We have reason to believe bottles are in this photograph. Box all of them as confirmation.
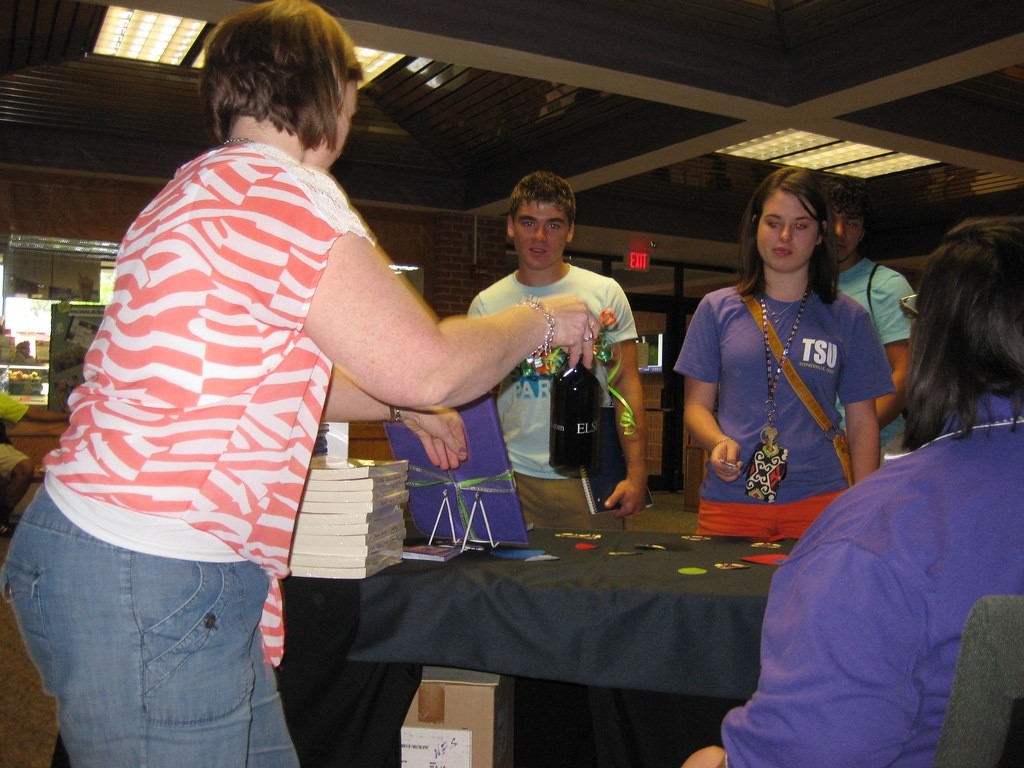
[550,346,601,471]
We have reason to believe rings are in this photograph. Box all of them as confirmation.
[584,330,593,342]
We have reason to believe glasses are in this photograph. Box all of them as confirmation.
[898,294,920,319]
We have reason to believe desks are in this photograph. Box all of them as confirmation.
[47,528,802,768]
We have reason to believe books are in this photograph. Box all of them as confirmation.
[580,406,652,515]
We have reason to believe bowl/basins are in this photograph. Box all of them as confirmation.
[9,379,42,396]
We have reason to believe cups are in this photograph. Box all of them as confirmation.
[36,340,49,361]
[0,334,16,362]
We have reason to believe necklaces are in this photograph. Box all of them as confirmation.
[764,291,804,324]
[222,138,254,145]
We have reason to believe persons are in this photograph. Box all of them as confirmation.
[681,218,1024,768]
[820,177,917,432]
[674,167,896,539]
[0,389,69,538]
[0,0,599,768]
[465,173,652,532]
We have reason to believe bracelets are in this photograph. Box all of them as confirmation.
[710,438,735,455]
[389,405,400,426]
[517,300,554,359]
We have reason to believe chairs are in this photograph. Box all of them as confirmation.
[930,594,1024,768]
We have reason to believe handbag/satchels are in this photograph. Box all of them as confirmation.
[829,428,855,490]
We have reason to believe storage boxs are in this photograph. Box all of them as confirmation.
[7,378,41,394]
[401,666,515,768]
